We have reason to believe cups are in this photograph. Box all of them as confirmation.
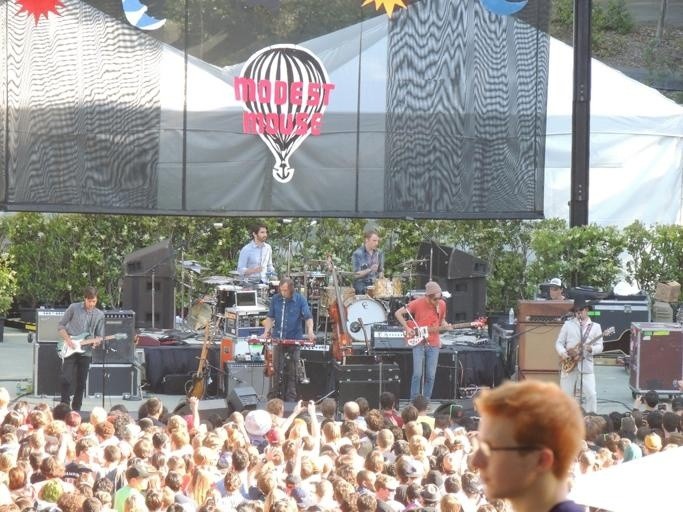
[317,265,321,274]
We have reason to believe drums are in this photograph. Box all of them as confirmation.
[343,293,389,343]
[291,270,326,290]
[368,277,403,299]
[215,284,243,305]
[191,301,214,329]
[320,286,355,318]
[268,280,281,295]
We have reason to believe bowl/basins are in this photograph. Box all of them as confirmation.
[250,344,263,355]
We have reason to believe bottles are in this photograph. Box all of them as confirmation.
[383,277,391,297]
[374,271,386,297]
[366,285,375,298]
[391,275,401,297]
[175,314,183,324]
[508,307,514,324]
[264,271,277,282]
[15,381,21,395]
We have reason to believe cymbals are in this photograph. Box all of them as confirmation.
[180,260,214,272]
[395,259,429,266]
[199,275,233,284]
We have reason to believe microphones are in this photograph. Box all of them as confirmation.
[578,316,584,326]
[101,302,107,309]
[282,233,296,240]
[395,299,408,307]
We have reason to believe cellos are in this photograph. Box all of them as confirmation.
[323,251,353,361]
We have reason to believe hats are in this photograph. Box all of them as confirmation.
[643,432,663,451]
[619,417,637,434]
[567,294,593,313]
[539,277,567,291]
[245,409,273,436]
[424,281,443,296]
[125,464,156,480]
[622,443,642,464]
[419,484,441,503]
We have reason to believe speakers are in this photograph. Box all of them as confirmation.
[125,239,176,276]
[91,309,136,365]
[122,276,175,329]
[417,280,487,324]
[372,347,413,399]
[36,308,67,344]
[295,344,335,401]
[416,242,489,280]
[430,351,457,402]
[223,361,274,402]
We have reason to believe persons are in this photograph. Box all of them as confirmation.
[554,295,604,413]
[0,378,683,512]
[237,224,278,286]
[548,278,566,300]
[351,222,384,295]
[394,282,446,407]
[263,279,315,401]
[57,288,105,410]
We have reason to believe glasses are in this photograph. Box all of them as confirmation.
[382,487,398,493]
[473,433,542,457]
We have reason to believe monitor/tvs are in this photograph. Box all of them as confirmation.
[235,290,258,307]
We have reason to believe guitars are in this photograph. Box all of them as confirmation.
[406,317,489,348]
[558,326,616,372]
[57,334,128,360]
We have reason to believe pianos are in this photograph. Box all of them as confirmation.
[516,299,574,373]
[249,338,315,347]
